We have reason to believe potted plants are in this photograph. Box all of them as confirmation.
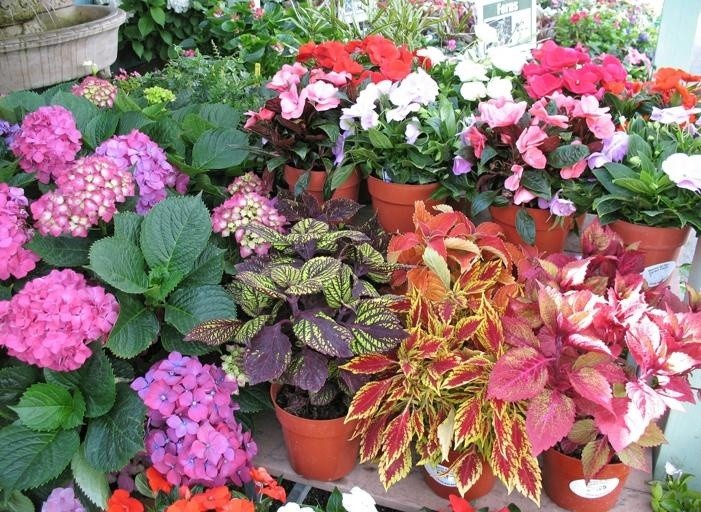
[171,198,413,481]
[483,218,701,512]
[340,199,543,507]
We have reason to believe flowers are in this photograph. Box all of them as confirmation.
[241,61,358,201]
[338,67,472,184]
[586,107,701,230]
[450,91,616,246]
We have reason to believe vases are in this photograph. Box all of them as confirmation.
[490,206,566,259]
[283,165,359,208]
[367,174,447,232]
[576,213,689,268]
